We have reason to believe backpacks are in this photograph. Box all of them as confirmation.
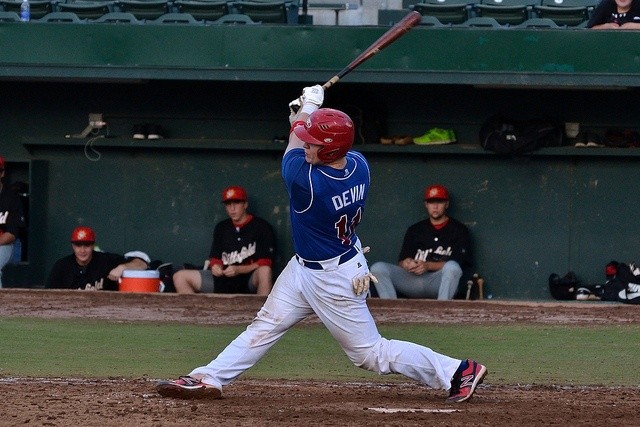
[549,269,593,299]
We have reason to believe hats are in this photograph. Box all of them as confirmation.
[423,185,449,201]
[221,186,247,202]
[70,227,95,243]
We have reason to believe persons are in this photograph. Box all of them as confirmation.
[155,83,487,403]
[171,184,273,293]
[0,155,22,275]
[585,0,640,30]
[44,226,148,291]
[369,184,472,302]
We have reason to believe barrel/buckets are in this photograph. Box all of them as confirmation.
[117,270,160,292]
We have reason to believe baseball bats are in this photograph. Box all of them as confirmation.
[202,260,211,271]
[289,11,423,115]
[466,280,474,301]
[472,273,479,301]
[476,278,486,299]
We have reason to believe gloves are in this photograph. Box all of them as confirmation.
[353,273,379,295]
[363,246,370,254]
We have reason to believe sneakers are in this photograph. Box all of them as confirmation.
[448,130,455,142]
[573,132,587,147]
[148,122,169,140]
[445,357,488,403]
[132,122,147,139]
[155,375,222,400]
[395,135,412,146]
[587,134,606,147]
[380,135,398,144]
[412,128,451,146]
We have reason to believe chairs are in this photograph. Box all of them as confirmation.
[451,17,509,29]
[415,15,452,28]
[510,18,567,29]
[145,12,205,26]
[205,13,262,25]
[87,12,145,25]
[0,11,20,22]
[30,11,88,24]
[568,19,590,29]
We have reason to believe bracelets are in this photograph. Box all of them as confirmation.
[289,121,308,135]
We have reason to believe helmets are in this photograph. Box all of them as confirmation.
[293,108,355,162]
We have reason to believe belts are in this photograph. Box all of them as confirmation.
[295,245,360,269]
